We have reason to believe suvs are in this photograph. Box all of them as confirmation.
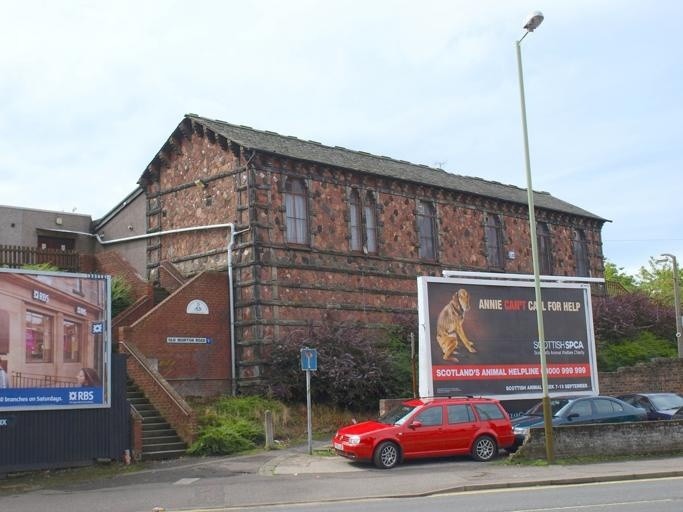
[332,394,513,469]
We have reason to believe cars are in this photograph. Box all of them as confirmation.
[614,392,683,421]
[505,394,649,452]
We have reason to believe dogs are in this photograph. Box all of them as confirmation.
[436,288,477,363]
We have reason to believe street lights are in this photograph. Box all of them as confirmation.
[656,252,683,360]
[513,10,554,465]
